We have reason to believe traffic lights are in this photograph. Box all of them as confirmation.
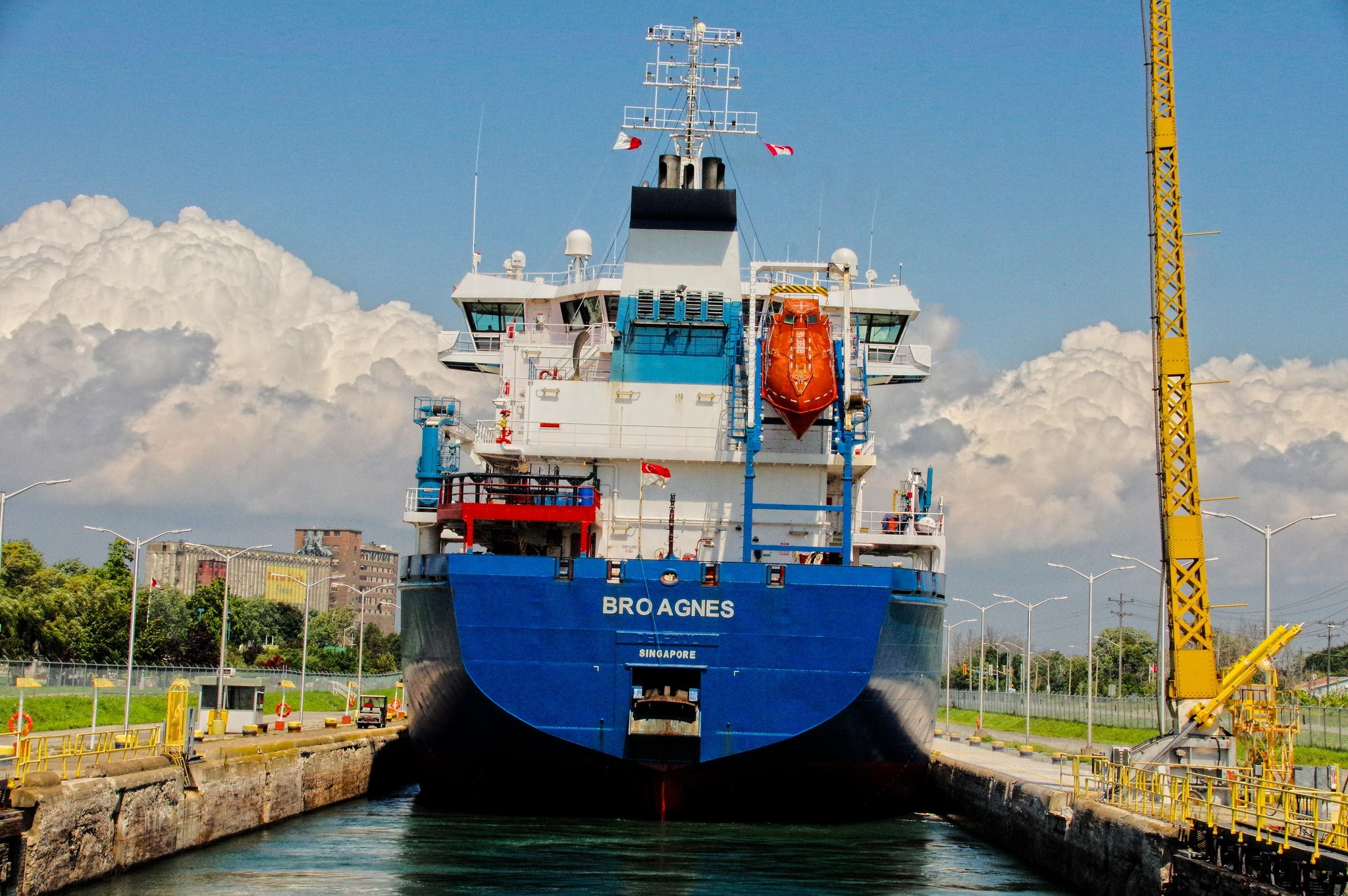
[963,664,966,675]
[1002,665,1005,673]
[1010,666,1013,677]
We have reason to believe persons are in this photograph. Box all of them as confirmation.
[487,325,495,331]
[364,699,374,707]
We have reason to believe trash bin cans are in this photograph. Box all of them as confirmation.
[545,496,555,506]
[566,492,573,506]
[578,487,594,505]
[556,493,566,506]
[534,496,541,505]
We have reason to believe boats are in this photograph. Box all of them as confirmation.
[761,297,838,440]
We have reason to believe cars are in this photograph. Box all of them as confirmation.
[356,695,388,729]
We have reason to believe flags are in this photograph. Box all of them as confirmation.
[613,131,643,150]
[642,463,672,488]
[152,577,161,589]
[765,142,794,156]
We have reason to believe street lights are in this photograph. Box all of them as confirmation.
[0,478,72,533]
[1030,652,1051,695]
[1201,508,1338,705]
[332,582,395,710]
[995,643,1009,693]
[82,525,192,734]
[992,592,1069,746]
[183,541,274,720]
[1069,645,1099,697]
[344,626,356,647]
[272,571,348,723]
[1004,641,1025,693]
[1094,636,1122,699]
[1047,562,1136,749]
[1109,552,1220,735]
[1050,649,1072,695]
[1020,654,1039,693]
[943,618,980,735]
[950,597,1015,732]
[982,643,1000,693]
[380,601,405,711]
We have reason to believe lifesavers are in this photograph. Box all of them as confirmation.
[390,698,402,709]
[976,716,980,730]
[275,702,291,717]
[349,695,355,708]
[7,712,33,736]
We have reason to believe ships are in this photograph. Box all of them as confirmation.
[397,15,948,810]
